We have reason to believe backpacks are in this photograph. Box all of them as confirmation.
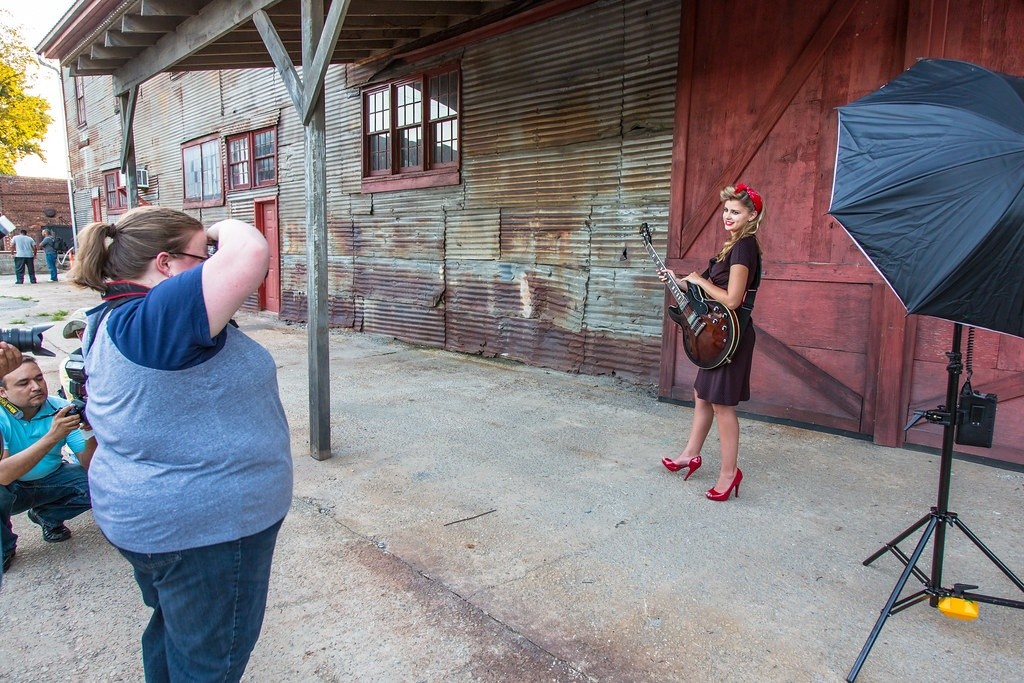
[47,236,63,250]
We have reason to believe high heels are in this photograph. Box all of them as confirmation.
[705,467,743,501]
[662,456,702,481]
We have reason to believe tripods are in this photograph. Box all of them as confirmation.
[845,322,1024,683]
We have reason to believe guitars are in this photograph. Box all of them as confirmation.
[637,219,741,370]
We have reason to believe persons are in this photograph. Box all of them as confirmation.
[67,205,293,683]
[11,230,37,283]
[656,183,764,501]
[0,307,98,574]
[40,228,61,282]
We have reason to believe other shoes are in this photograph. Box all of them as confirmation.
[31,282,37,284]
[47,279,58,283]
[15,282,23,284]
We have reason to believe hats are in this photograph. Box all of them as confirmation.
[63,306,96,339]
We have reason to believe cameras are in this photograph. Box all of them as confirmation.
[65,360,89,426]
[0,326,56,357]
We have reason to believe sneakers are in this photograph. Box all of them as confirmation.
[0,549,16,573]
[28,510,71,541]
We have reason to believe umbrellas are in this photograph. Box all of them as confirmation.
[826,59,1024,683]
